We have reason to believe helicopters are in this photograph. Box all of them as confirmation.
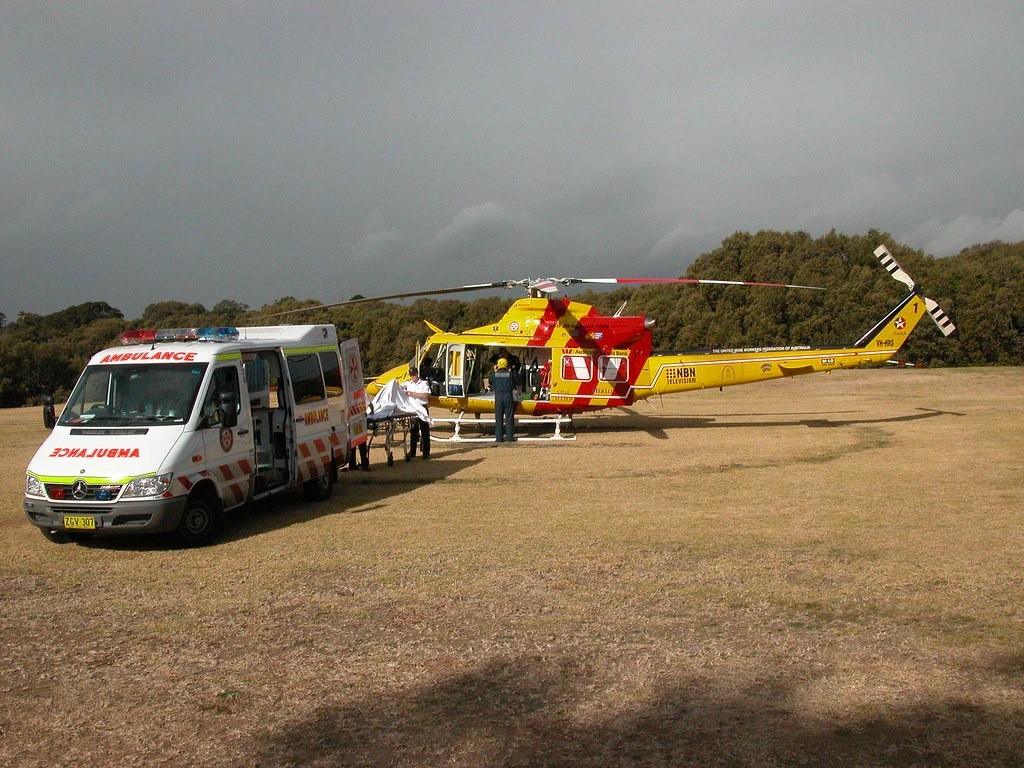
[244,238,956,446]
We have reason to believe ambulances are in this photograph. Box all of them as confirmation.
[21,323,370,548]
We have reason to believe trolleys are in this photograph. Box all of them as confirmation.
[365,408,426,467]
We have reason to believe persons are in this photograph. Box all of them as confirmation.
[401,368,432,459]
[489,347,521,442]
[349,393,375,471]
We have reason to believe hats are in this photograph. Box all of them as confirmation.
[409,367,418,374]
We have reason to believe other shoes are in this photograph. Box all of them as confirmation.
[495,440,504,442]
[508,438,517,441]
[422,454,430,459]
[407,453,415,457]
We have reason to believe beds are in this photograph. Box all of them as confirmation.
[366,412,418,465]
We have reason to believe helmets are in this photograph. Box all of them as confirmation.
[497,358,509,369]
